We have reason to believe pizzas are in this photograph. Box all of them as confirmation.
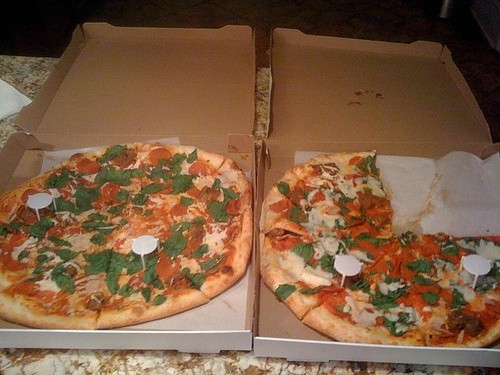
[259,150,500,348]
[0,141,252,330]
